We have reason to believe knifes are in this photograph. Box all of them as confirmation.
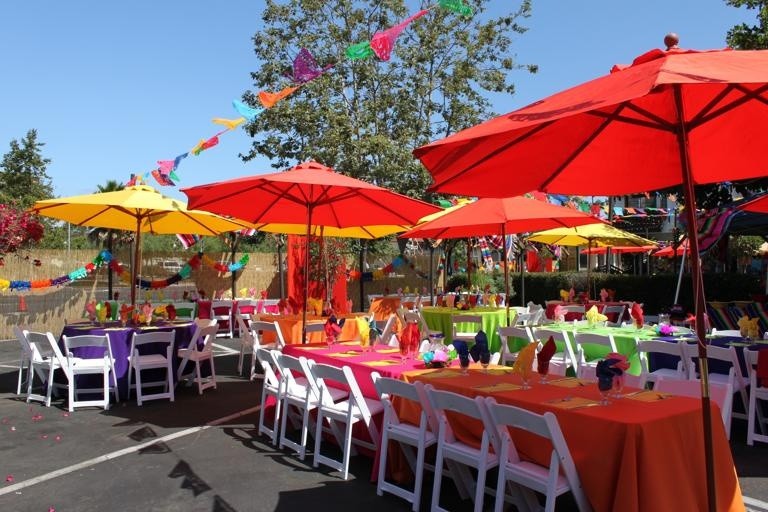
[627,387,651,397]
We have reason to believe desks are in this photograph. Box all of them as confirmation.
[259,311,369,328]
[372,293,443,304]
[199,300,280,319]
[544,299,633,312]
[445,291,506,301]
[510,321,692,346]
[399,362,716,436]
[420,305,516,321]
[65,320,195,336]
[651,334,767,352]
[281,343,476,384]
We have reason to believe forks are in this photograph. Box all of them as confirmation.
[656,393,680,401]
[548,395,572,405]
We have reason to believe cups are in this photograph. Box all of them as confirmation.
[740,326,749,343]
[748,329,757,348]
[325,336,334,351]
[121,315,127,327]
[359,334,369,353]
[144,314,152,326]
[408,341,419,363]
[520,364,532,391]
[480,351,491,375]
[89,311,96,325]
[398,343,411,366]
[537,362,551,384]
[611,374,623,398]
[457,354,472,377]
[597,377,610,406]
[370,333,376,351]
[552,314,560,328]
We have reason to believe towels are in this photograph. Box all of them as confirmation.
[421,285,428,298]
[166,303,177,321]
[514,341,540,379]
[240,288,249,296]
[468,292,478,307]
[143,304,153,323]
[630,303,644,325]
[749,318,758,337]
[489,295,496,308]
[585,305,608,327]
[551,305,567,322]
[569,288,574,301]
[248,287,256,296]
[452,339,471,368]
[594,359,621,390]
[355,314,370,348]
[330,316,345,338]
[118,303,132,322]
[171,290,180,302]
[144,289,152,303]
[214,287,226,303]
[261,290,268,299]
[403,285,411,295]
[369,320,378,345]
[322,321,333,342]
[85,301,97,322]
[738,316,750,335]
[98,306,108,323]
[560,290,568,303]
[413,287,419,297]
[157,290,166,302]
[538,335,557,374]
[495,294,503,306]
[445,293,457,307]
[599,288,607,303]
[470,331,491,366]
[105,302,112,319]
[395,287,402,296]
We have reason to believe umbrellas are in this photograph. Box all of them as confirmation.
[415,201,483,293]
[31,178,247,313]
[525,224,659,301]
[178,159,446,344]
[736,190,767,215]
[227,214,416,314]
[651,237,701,272]
[397,197,613,329]
[412,33,768,512]
[579,245,658,273]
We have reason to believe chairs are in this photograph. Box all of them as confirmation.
[400,296,419,313]
[175,323,220,396]
[368,294,384,305]
[402,309,444,343]
[637,340,685,392]
[63,332,120,413]
[498,326,535,365]
[261,299,281,315]
[420,296,433,307]
[370,371,467,511]
[532,360,567,376]
[559,305,585,321]
[22,329,76,408]
[233,299,258,338]
[306,324,326,331]
[744,346,768,446]
[456,351,501,364]
[256,349,325,445]
[575,363,647,392]
[486,394,593,512]
[424,382,530,512]
[236,314,253,377]
[306,358,386,478]
[531,326,577,375]
[711,327,742,338]
[651,375,733,441]
[127,330,175,406]
[210,299,233,339]
[380,313,397,345]
[174,302,196,323]
[273,349,350,461]
[249,320,285,384]
[13,326,60,398]
[603,305,626,326]
[682,340,752,421]
[451,314,483,342]
[572,329,619,381]
